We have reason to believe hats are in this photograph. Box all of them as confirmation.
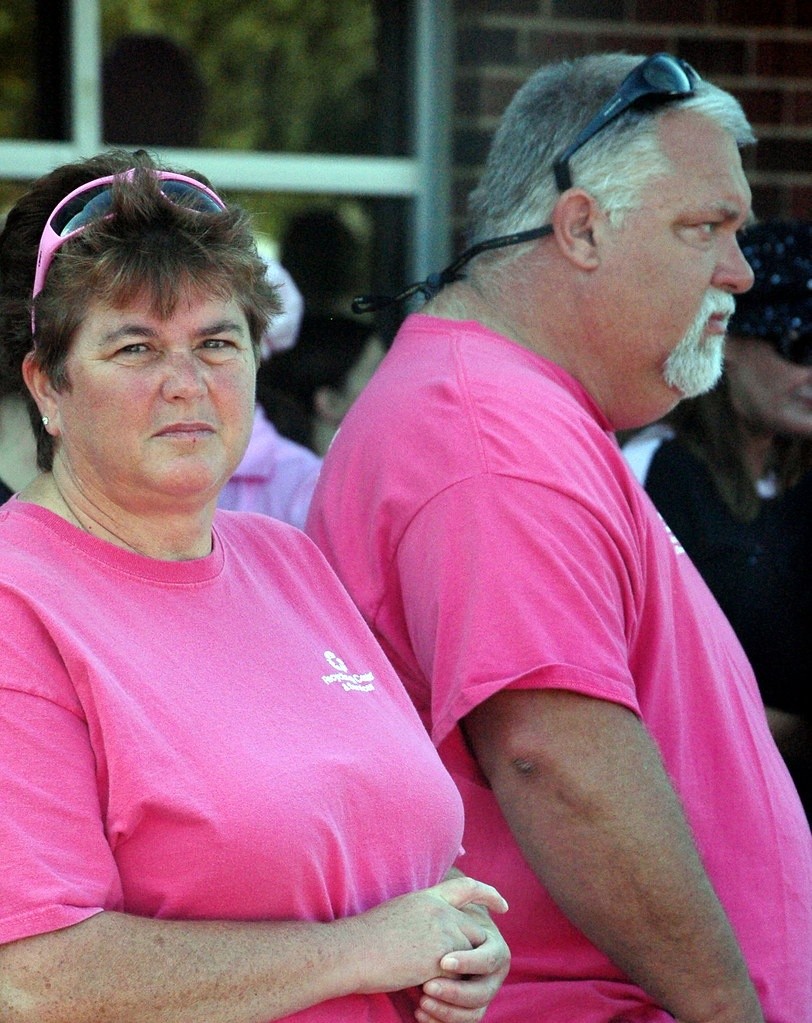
[727,220,812,341]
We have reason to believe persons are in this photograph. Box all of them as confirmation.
[0,147,513,1023]
[303,49,812,1023]
[3,34,812,829]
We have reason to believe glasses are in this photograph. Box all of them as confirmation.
[767,337,812,366]
[32,168,231,349]
[553,54,700,196]
